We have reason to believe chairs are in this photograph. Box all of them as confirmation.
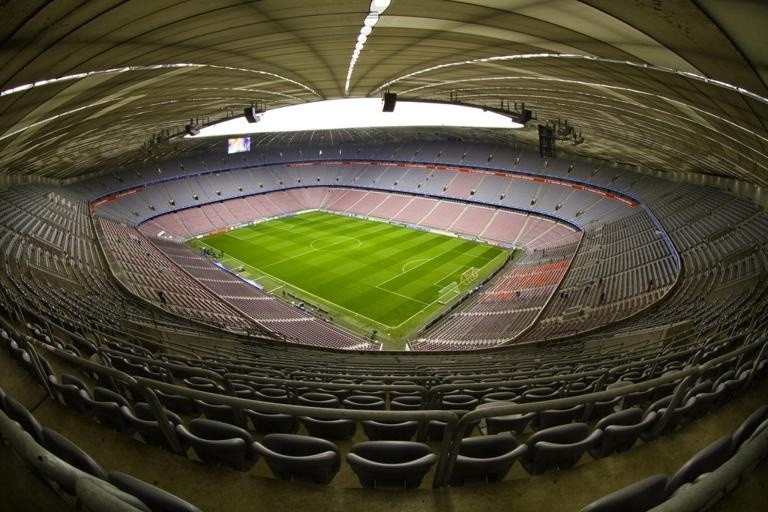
[0,140,768,511]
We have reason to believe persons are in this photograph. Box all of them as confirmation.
[157,289,167,306]
[645,279,653,294]
[204,247,224,260]
[515,290,522,298]
[146,252,149,257]
[282,289,285,298]
[137,240,141,245]
[557,276,606,305]
